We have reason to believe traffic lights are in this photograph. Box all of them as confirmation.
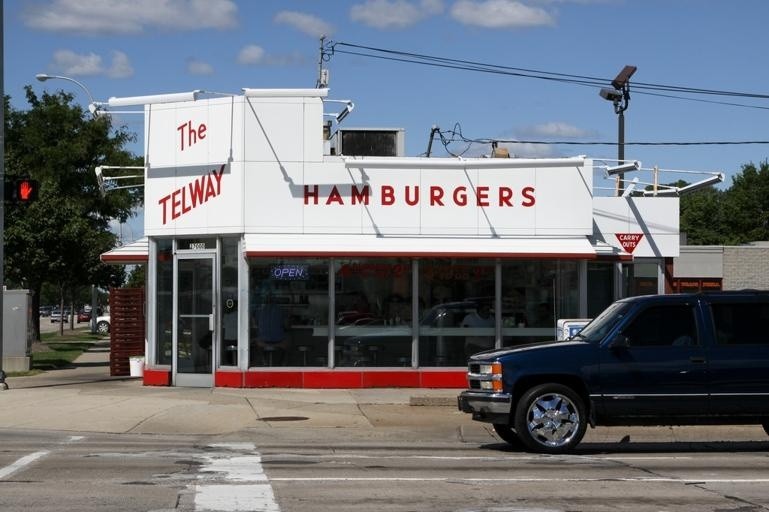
[18,179,36,199]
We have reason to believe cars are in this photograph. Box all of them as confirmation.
[77,304,103,324]
[337,297,528,367]
[38,304,74,324]
[88,316,110,333]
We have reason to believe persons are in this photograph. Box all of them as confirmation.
[459,301,496,362]
[222,302,288,363]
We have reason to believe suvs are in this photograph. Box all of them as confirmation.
[456,291,769,453]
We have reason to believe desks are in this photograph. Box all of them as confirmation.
[310,327,556,339]
[250,324,314,329]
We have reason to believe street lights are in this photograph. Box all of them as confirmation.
[36,72,94,105]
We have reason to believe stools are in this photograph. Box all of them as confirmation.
[208,344,449,366]
